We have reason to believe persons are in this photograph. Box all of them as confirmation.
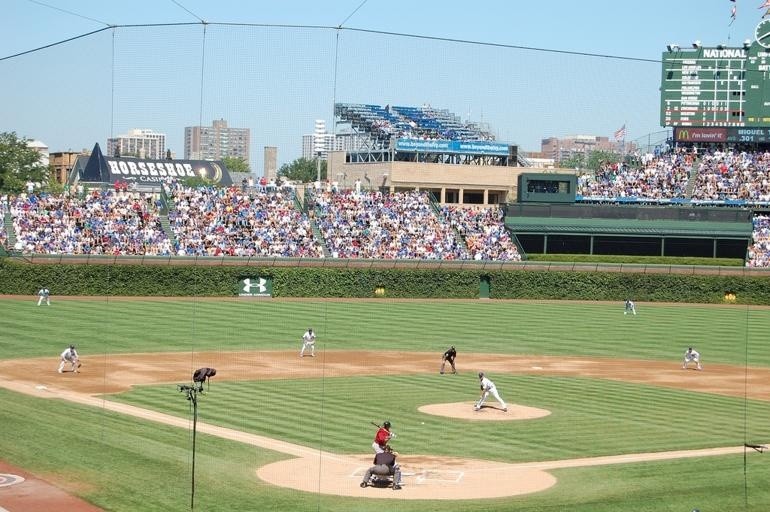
[473,372,507,411]
[1,175,522,261]
[440,345,458,374]
[372,104,496,141]
[623,299,636,315]
[37,287,50,306]
[683,348,700,369]
[300,327,317,359]
[57,345,80,374]
[360,421,403,490]
[576,142,769,266]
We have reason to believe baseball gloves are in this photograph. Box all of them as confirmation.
[392,452,398,456]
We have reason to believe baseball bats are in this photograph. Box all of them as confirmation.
[371,421,394,436]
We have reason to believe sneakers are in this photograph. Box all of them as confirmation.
[393,485,401,490]
[360,483,366,488]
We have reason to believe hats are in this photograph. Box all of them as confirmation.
[382,446,393,450]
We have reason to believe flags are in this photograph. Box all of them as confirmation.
[614,125,626,142]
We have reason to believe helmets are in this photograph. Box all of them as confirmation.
[384,421,391,428]
